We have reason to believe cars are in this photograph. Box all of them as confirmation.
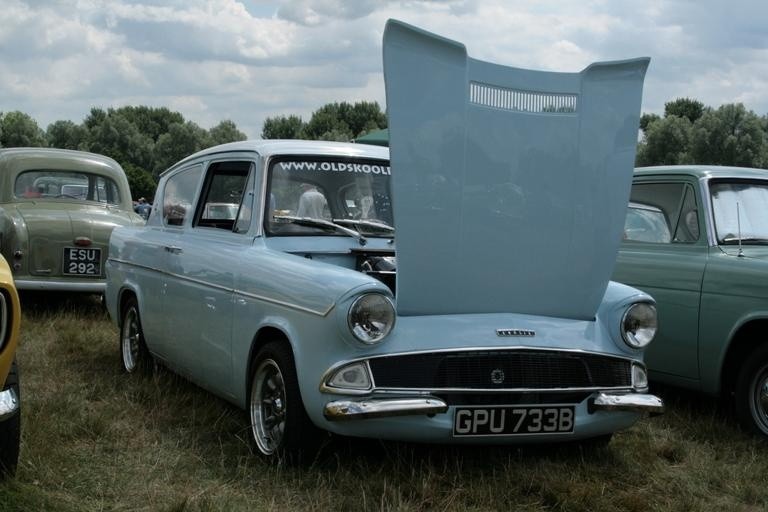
[0,147,147,294]
[611,161,767,440]
[104,16,664,468]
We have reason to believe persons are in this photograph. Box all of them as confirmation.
[295,182,327,222]
[12,180,293,225]
